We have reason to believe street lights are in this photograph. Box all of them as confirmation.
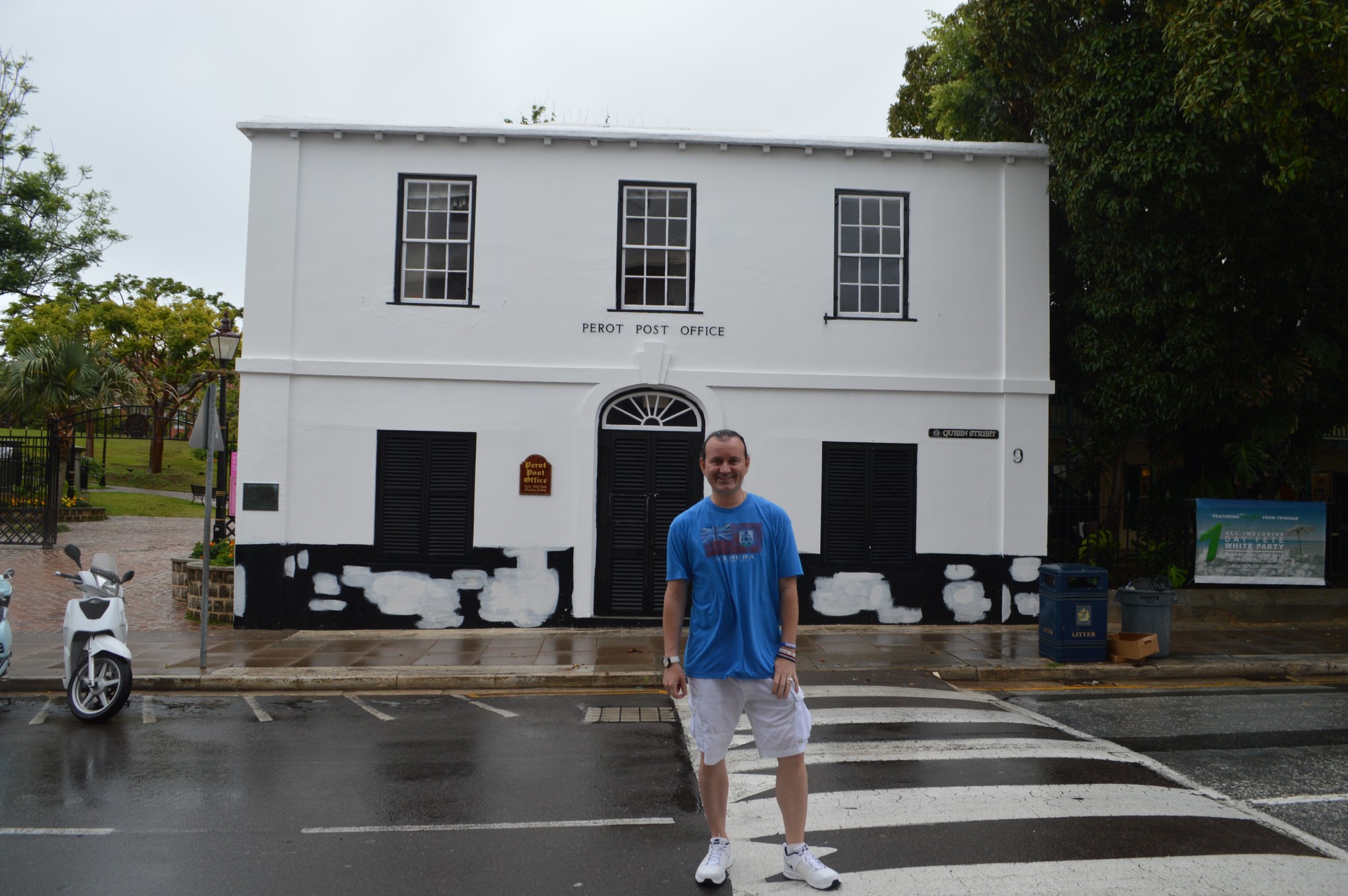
[208,308,245,544]
[99,398,115,486]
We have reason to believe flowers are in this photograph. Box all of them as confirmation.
[1,495,93,508]
[191,537,235,566]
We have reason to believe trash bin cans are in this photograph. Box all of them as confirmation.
[1115,586,1175,659]
[1038,563,1111,662]
[0,440,25,486]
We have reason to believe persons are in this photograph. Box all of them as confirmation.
[662,429,840,892]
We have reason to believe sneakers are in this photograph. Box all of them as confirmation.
[695,834,735,883]
[784,842,841,891]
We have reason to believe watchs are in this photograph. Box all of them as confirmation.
[662,656,680,667]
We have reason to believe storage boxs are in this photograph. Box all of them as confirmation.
[1107,632,1160,663]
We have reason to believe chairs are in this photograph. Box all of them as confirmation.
[190,484,229,505]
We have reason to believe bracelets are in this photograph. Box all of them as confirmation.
[780,642,796,648]
[777,648,797,663]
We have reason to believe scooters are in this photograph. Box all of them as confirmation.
[0,568,16,703]
[55,544,135,724]
